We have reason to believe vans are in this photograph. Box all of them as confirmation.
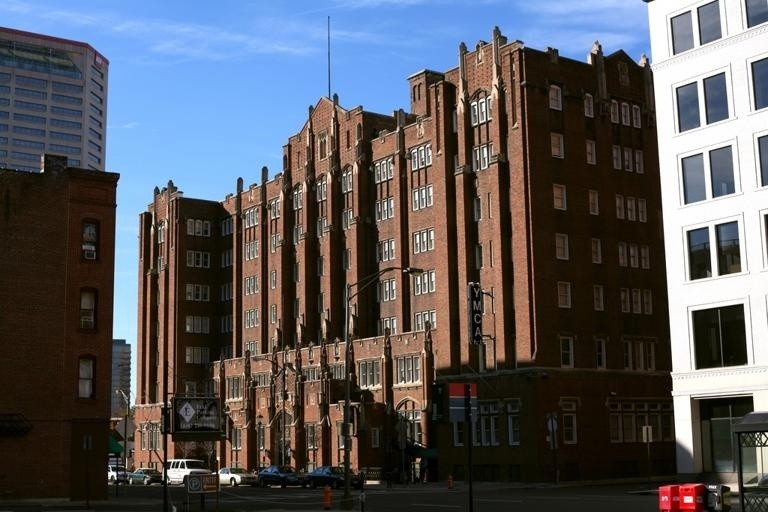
[161,458,213,485]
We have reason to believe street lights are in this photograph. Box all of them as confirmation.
[341,267,423,512]
[253,357,285,467]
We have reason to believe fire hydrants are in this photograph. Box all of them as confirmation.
[448,475,453,489]
[323,484,333,510]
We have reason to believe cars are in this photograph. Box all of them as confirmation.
[212,467,259,487]
[258,465,359,489]
[108,465,162,485]
